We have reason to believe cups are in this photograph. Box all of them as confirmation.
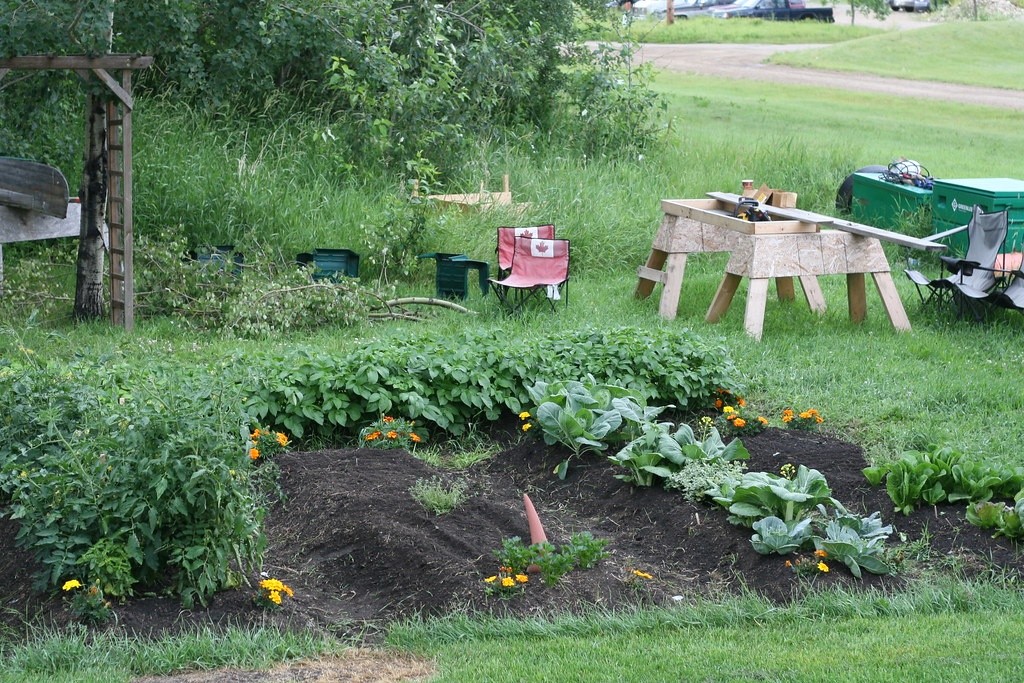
[741,180,753,199]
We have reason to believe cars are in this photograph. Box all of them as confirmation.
[889,0,930,13]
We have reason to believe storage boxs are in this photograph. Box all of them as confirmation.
[850,171,1024,261]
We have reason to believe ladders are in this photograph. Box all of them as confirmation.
[103,71,134,331]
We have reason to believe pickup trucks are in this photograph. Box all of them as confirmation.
[706,0,835,23]
[633,0,805,20]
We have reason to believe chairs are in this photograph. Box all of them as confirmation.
[494,224,556,309]
[418,252,490,304]
[903,203,1024,326]
[487,236,570,318]
[184,244,244,276]
[296,248,360,282]
